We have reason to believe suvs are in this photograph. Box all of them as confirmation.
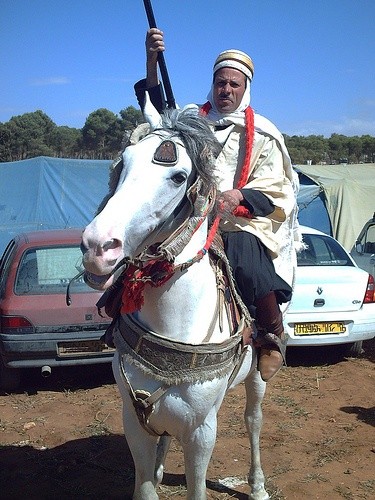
[349,212,375,277]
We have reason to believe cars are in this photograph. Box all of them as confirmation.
[1,229,115,378]
[280,225,375,356]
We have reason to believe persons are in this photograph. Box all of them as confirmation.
[101,27,295,383]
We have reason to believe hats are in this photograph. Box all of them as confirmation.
[212,49,254,83]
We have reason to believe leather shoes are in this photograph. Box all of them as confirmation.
[258,327,285,381]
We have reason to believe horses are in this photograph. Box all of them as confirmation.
[78,88,273,500]
